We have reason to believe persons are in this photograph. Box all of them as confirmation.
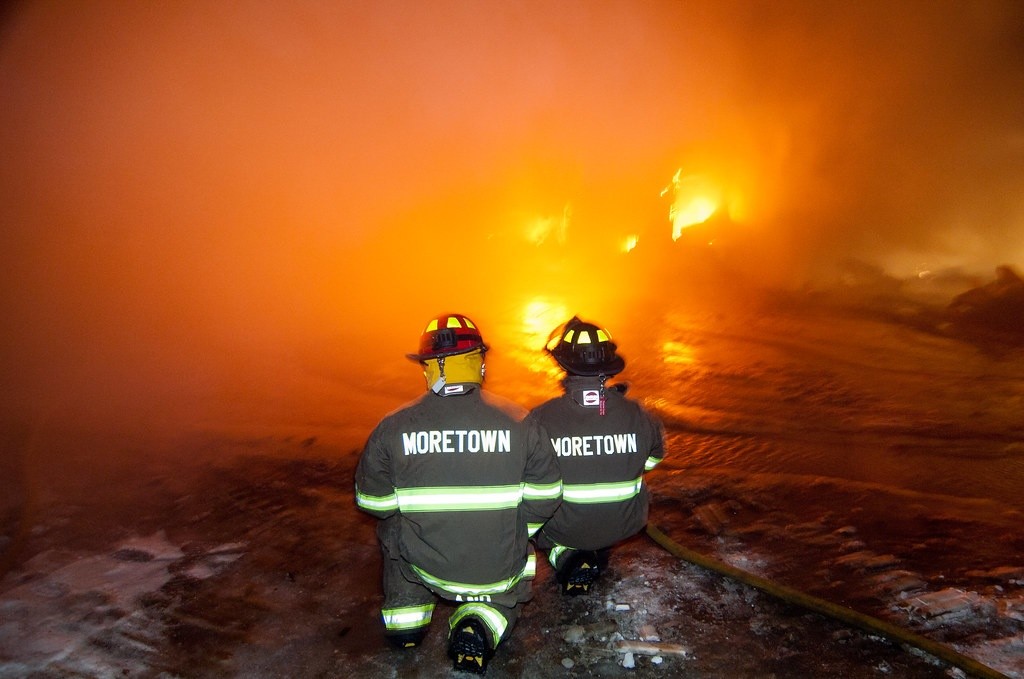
[352,313,564,678]
[522,314,665,588]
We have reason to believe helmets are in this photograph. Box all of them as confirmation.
[404,314,491,363]
[551,323,625,377]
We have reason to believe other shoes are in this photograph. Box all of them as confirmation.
[560,546,609,593]
[389,626,429,651]
[447,617,488,674]
